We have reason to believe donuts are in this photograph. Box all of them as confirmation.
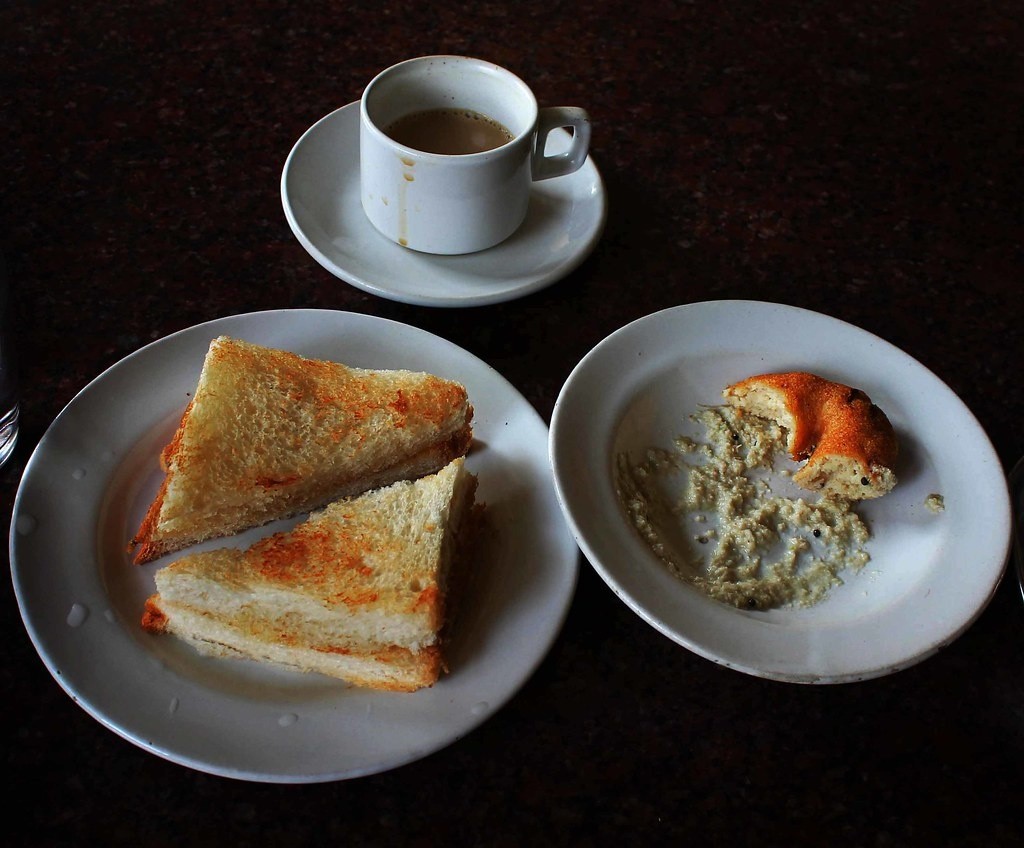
[720,371,900,502]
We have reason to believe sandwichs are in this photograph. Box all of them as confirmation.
[128,338,476,692]
